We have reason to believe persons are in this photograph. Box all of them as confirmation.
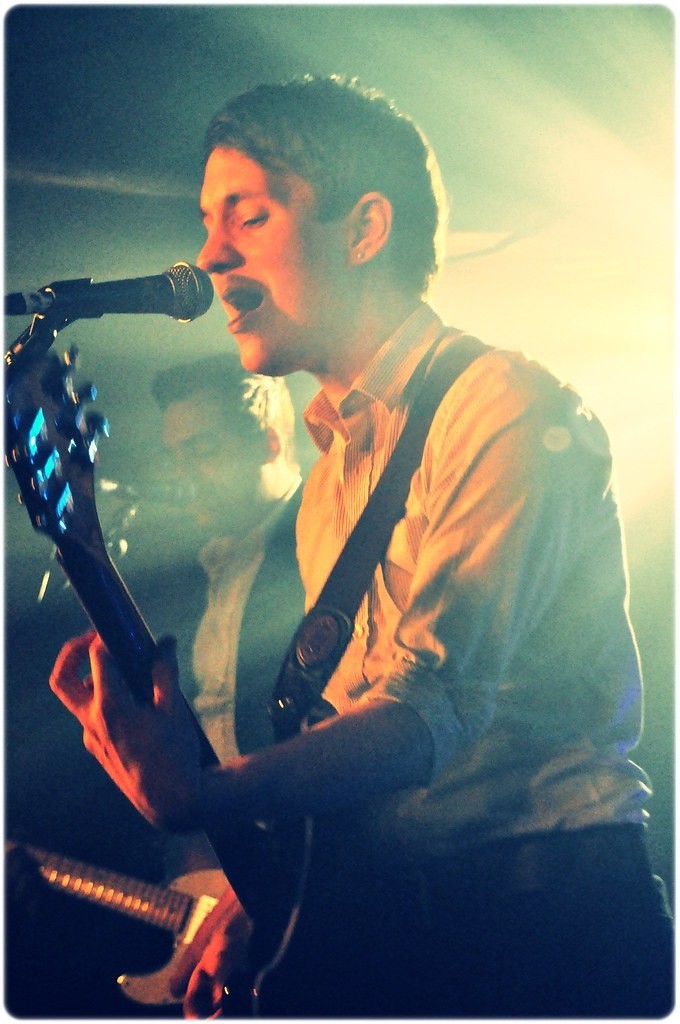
[50,71,675,1017]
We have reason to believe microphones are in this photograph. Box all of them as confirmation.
[4,261,214,324]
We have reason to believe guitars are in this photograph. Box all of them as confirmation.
[3,296,399,1020]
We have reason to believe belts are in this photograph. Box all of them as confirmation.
[348,827,649,904]
